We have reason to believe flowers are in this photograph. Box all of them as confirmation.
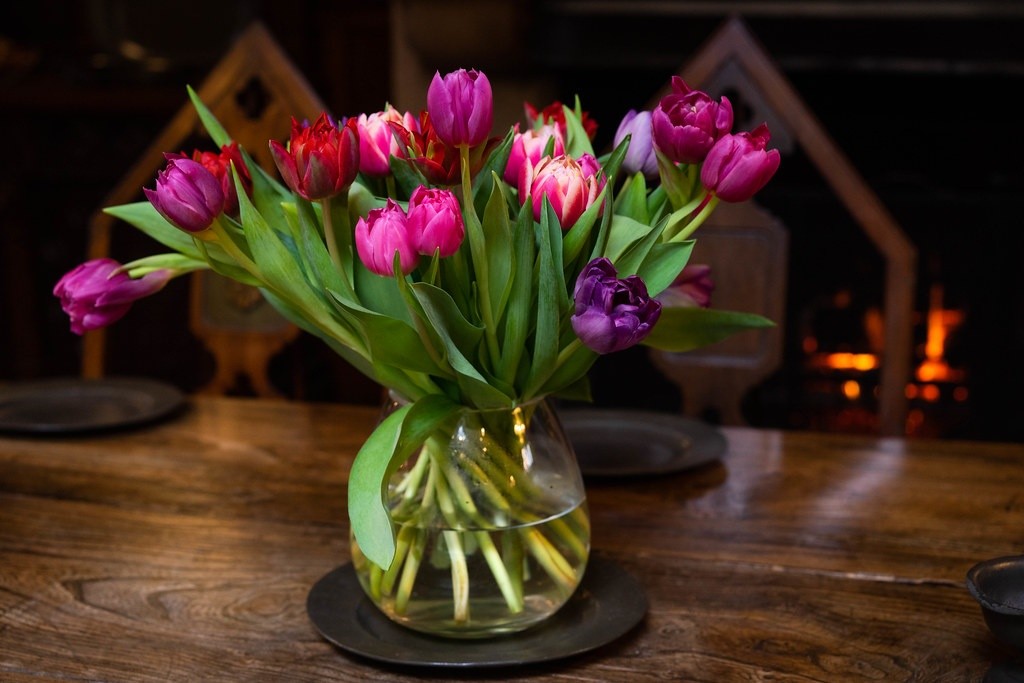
[51,67,785,625]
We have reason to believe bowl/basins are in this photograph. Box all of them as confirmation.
[966,554,1024,644]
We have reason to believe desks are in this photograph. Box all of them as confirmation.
[0,396,1024,683]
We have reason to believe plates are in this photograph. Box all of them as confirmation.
[550,408,728,477]
[303,550,647,670]
[0,380,187,436]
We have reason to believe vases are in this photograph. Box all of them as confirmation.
[349,399,594,641]
